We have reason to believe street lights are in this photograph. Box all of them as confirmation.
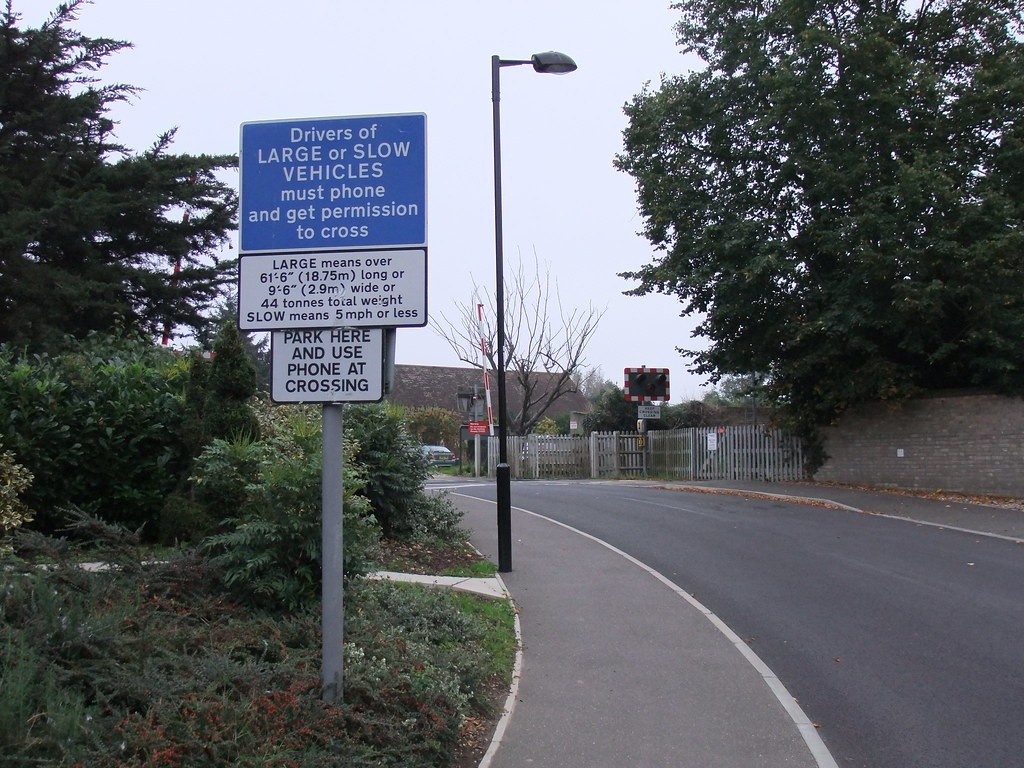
[490,50,580,573]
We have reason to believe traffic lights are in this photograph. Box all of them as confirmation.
[623,368,671,402]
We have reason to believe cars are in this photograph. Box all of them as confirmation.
[422,445,456,470]
